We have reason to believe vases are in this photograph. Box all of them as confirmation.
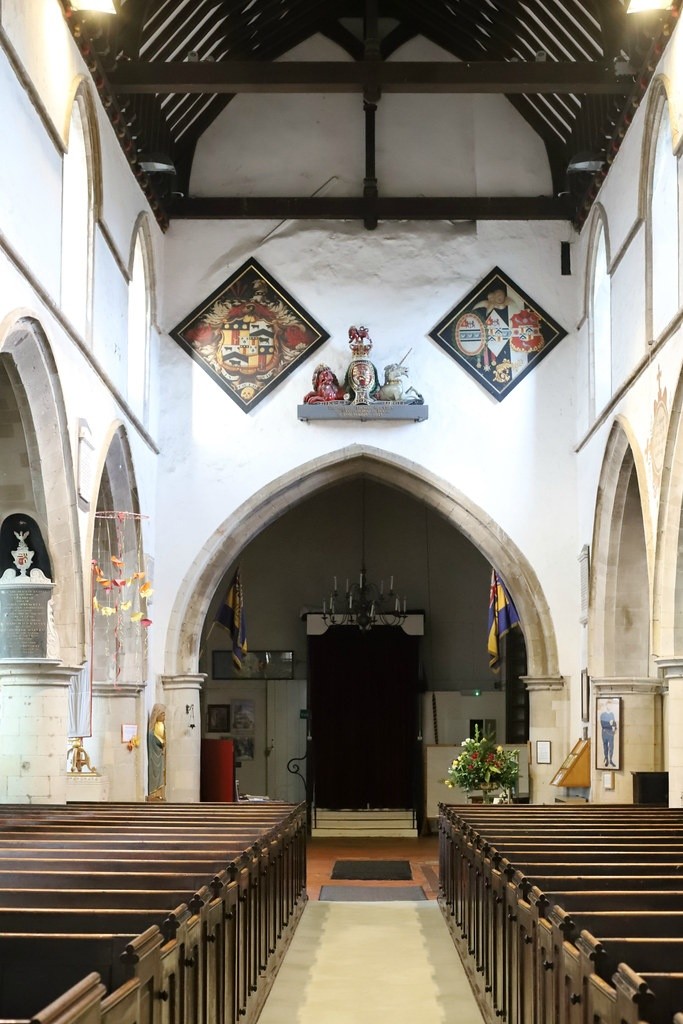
[483,788,493,804]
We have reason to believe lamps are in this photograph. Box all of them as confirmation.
[626,0,673,14]
[138,152,176,176]
[70,0,117,15]
[565,152,606,173]
[320,474,408,635]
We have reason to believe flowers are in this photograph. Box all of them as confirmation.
[438,724,523,793]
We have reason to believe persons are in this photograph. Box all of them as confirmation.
[147,703,166,801]
[600,701,617,768]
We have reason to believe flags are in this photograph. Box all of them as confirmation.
[213,562,249,671]
[488,567,520,673]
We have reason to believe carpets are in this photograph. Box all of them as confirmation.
[331,861,413,880]
[318,885,429,902]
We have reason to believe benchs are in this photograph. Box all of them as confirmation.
[436,802,683,1024]
[0,801,310,1024]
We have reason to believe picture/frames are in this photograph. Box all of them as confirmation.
[168,255,331,416]
[580,667,590,723]
[211,649,295,680]
[595,697,622,771]
[207,704,231,733]
[536,741,552,764]
[429,265,570,403]
